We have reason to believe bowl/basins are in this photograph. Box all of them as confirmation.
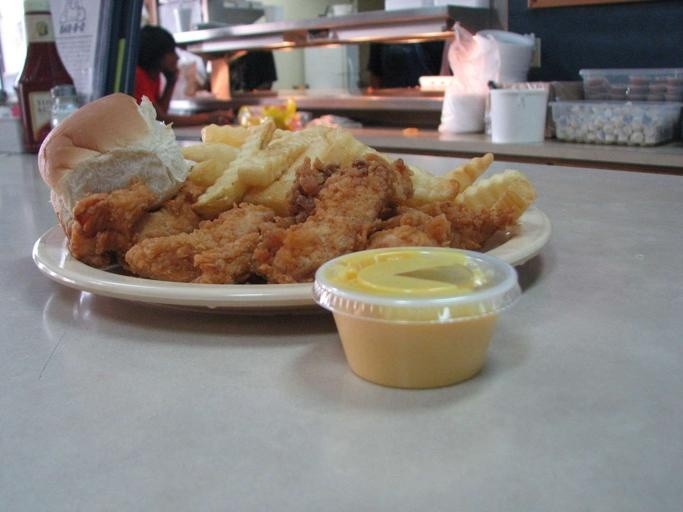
[222,7,263,26]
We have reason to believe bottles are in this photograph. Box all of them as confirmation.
[50,85,78,129]
[12,0,77,157]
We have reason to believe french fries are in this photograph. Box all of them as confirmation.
[182,118,537,223]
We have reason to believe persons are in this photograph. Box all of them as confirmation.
[200,49,277,91]
[128,23,235,129]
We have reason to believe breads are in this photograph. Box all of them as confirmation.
[36,93,191,238]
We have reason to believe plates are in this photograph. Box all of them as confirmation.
[30,201,550,318]
[272,44,360,93]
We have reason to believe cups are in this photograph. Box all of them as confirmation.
[174,7,192,33]
[313,243,522,391]
[332,5,352,17]
